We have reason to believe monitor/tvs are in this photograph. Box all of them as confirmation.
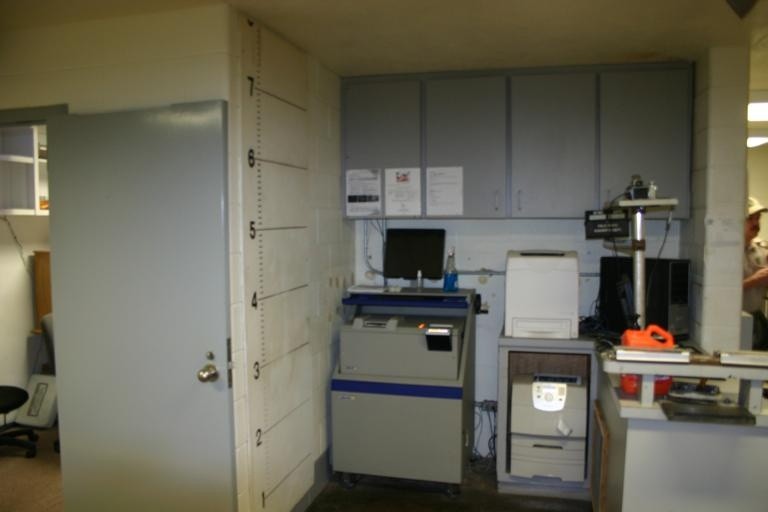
[381,227,445,282]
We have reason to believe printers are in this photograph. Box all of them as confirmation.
[509,370,588,483]
[503,249,580,339]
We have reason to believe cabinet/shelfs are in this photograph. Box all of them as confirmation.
[423,66,599,221]
[340,74,424,221]
[1,123,49,218]
[597,61,697,221]
[496,331,597,498]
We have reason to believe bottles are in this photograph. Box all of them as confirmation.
[443,245,459,293]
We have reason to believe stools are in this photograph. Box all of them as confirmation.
[1,384,41,461]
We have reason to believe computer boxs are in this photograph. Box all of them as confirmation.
[599,255,691,341]
